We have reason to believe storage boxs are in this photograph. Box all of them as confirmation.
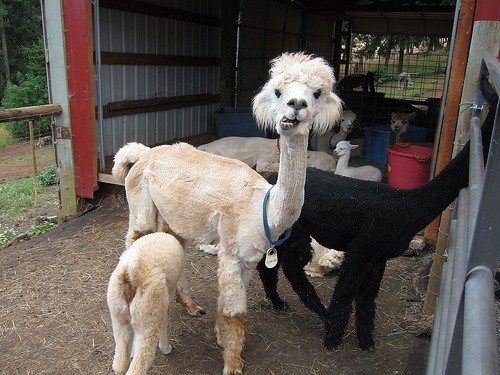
[337,91,386,110]
[363,123,427,166]
[214,105,270,138]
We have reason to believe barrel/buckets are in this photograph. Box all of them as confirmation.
[424,97,441,117]
[386,144,433,191]
